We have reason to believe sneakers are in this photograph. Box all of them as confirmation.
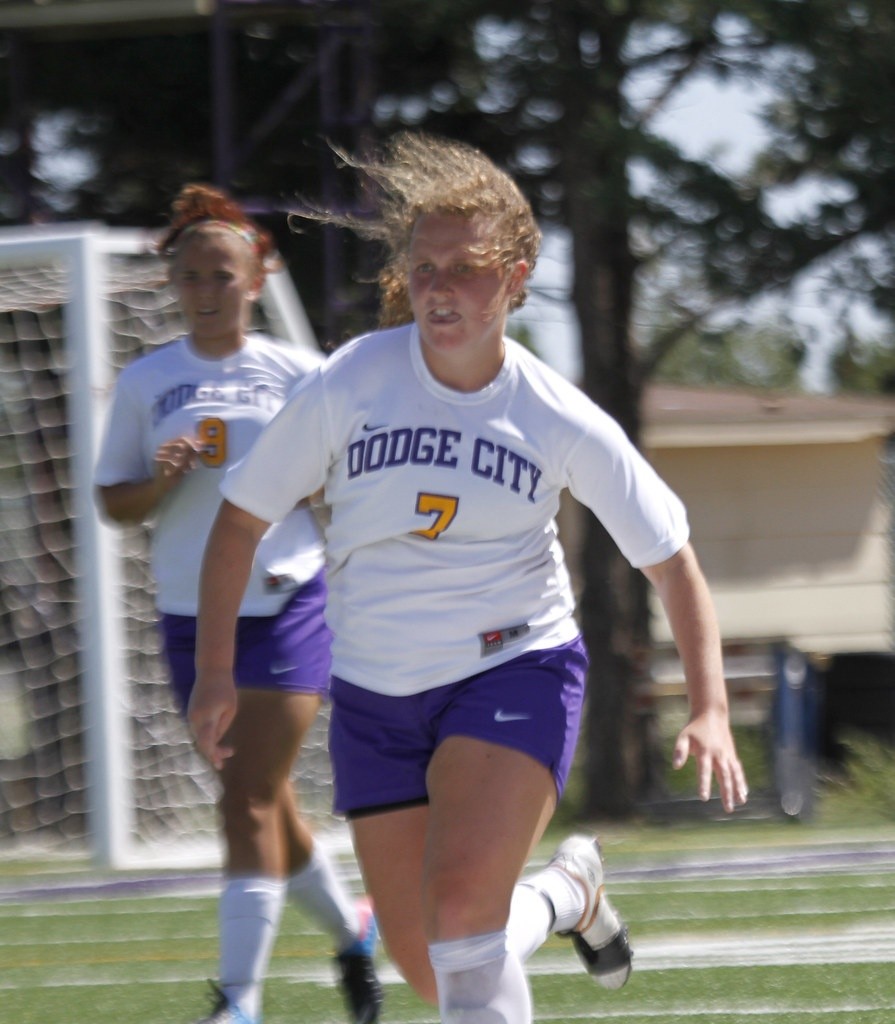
[553,834,634,992]
[196,982,263,1024]
[334,898,381,1023]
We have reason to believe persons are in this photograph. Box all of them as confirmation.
[188,146,748,1024]
[92,185,387,1024]
